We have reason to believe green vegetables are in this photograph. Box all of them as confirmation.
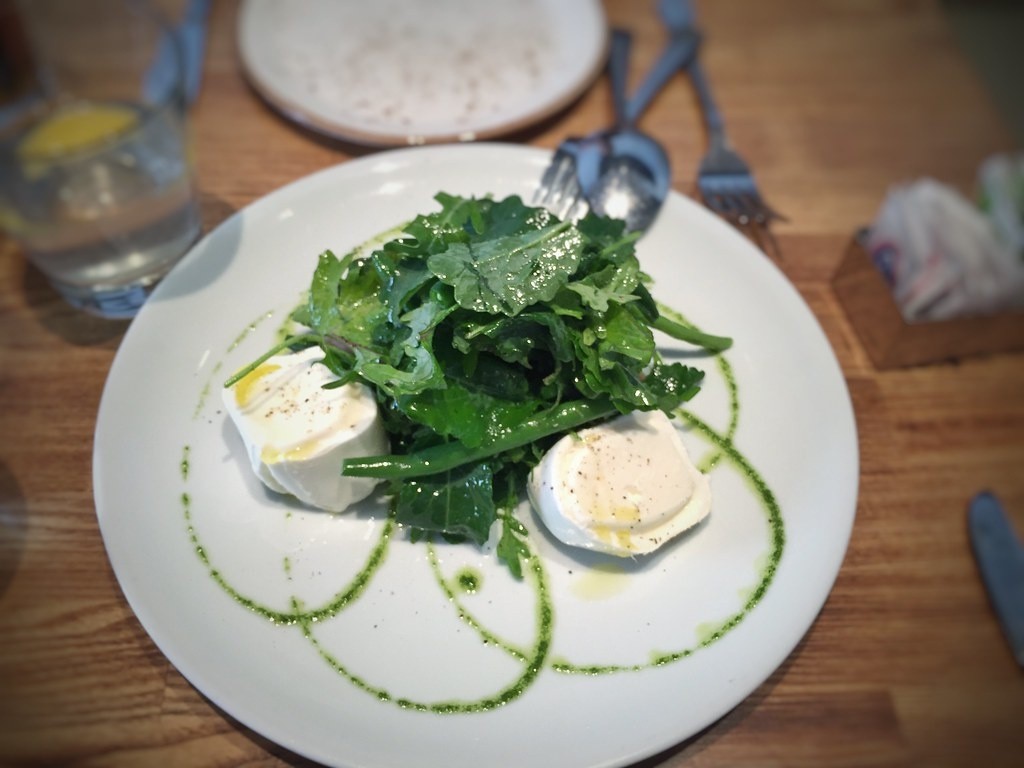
[221,192,732,577]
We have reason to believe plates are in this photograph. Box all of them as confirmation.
[239,0,607,142]
[93,144,860,768]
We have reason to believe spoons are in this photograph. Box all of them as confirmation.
[579,43,669,235]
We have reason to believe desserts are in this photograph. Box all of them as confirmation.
[221,346,393,514]
[526,391,712,557]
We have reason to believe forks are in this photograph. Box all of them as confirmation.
[536,30,700,220]
[660,0,792,229]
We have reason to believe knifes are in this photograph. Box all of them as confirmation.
[969,493,1024,671]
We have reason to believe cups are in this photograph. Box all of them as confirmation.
[0,0,201,314]
